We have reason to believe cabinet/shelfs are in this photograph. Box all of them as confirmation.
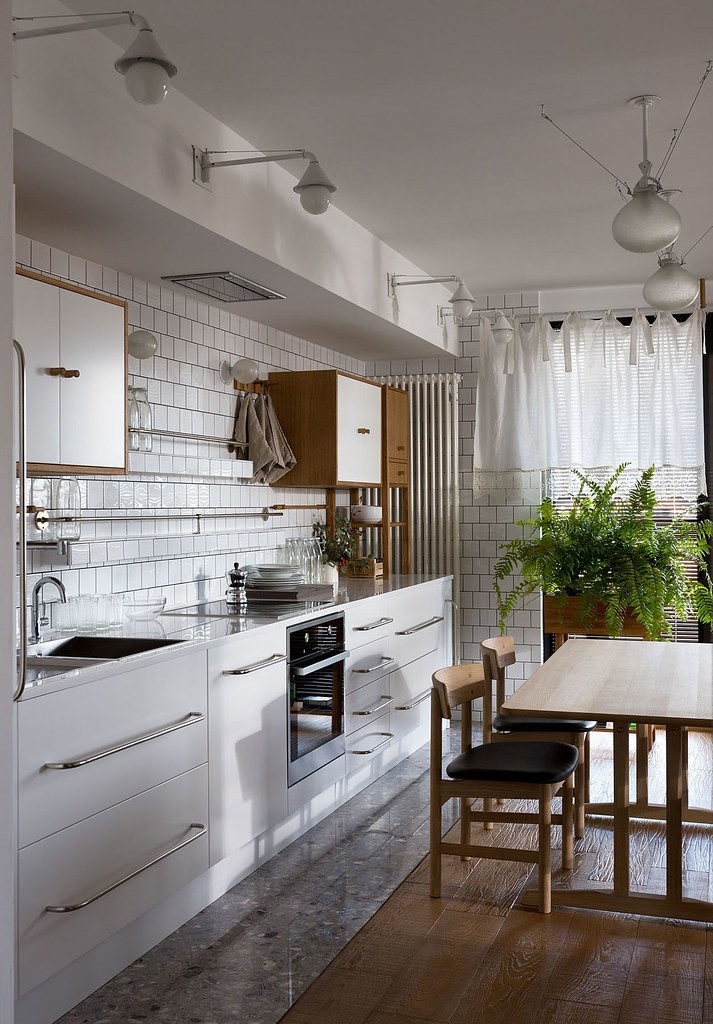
[269,370,384,490]
[14,264,130,477]
[13,625,294,1024]
[327,383,412,578]
[336,576,458,810]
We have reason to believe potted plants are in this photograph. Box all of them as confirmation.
[493,461,713,643]
[311,496,376,582]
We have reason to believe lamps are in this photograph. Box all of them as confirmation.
[540,60,713,253]
[219,358,260,386]
[617,181,713,312]
[192,144,337,215]
[12,10,177,105]
[387,272,475,320]
[436,304,516,344]
[128,331,157,360]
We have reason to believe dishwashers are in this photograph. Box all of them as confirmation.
[286,610,350,788]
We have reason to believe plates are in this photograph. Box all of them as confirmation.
[248,564,306,586]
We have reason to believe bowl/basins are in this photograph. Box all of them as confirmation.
[123,597,166,621]
[350,505,382,524]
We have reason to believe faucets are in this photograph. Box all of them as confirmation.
[27,576,68,643]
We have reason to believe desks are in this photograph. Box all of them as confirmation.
[501,638,713,921]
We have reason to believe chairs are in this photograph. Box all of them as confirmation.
[481,636,599,838]
[430,662,580,913]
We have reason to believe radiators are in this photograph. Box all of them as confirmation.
[349,371,465,667]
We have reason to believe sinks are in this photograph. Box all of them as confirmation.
[14,633,193,668]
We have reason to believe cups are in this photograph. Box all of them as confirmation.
[58,593,122,633]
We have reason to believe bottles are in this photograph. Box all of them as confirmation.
[285,538,323,584]
[55,476,81,541]
[225,563,248,604]
[132,388,152,453]
[127,385,139,450]
